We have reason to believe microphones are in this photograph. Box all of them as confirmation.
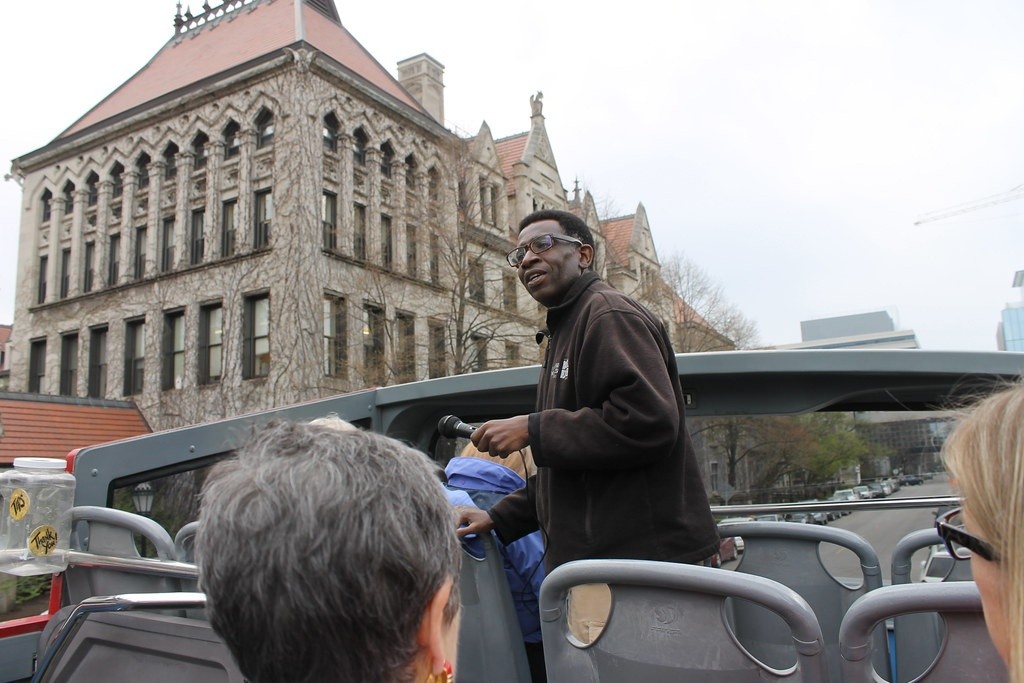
[438,414,478,439]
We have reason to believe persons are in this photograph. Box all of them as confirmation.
[440,441,548,683]
[940,383,1024,683]
[453,210,719,578]
[194,418,461,683]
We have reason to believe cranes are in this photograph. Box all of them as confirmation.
[912,180,1024,226]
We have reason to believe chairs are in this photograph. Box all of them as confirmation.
[26,489,1008,683]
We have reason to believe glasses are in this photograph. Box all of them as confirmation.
[506,233,583,267]
[936,507,1003,561]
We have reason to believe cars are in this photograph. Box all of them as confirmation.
[921,472,933,480]
[718,516,755,524]
[900,475,924,486]
[755,514,787,522]
[785,475,903,524]
